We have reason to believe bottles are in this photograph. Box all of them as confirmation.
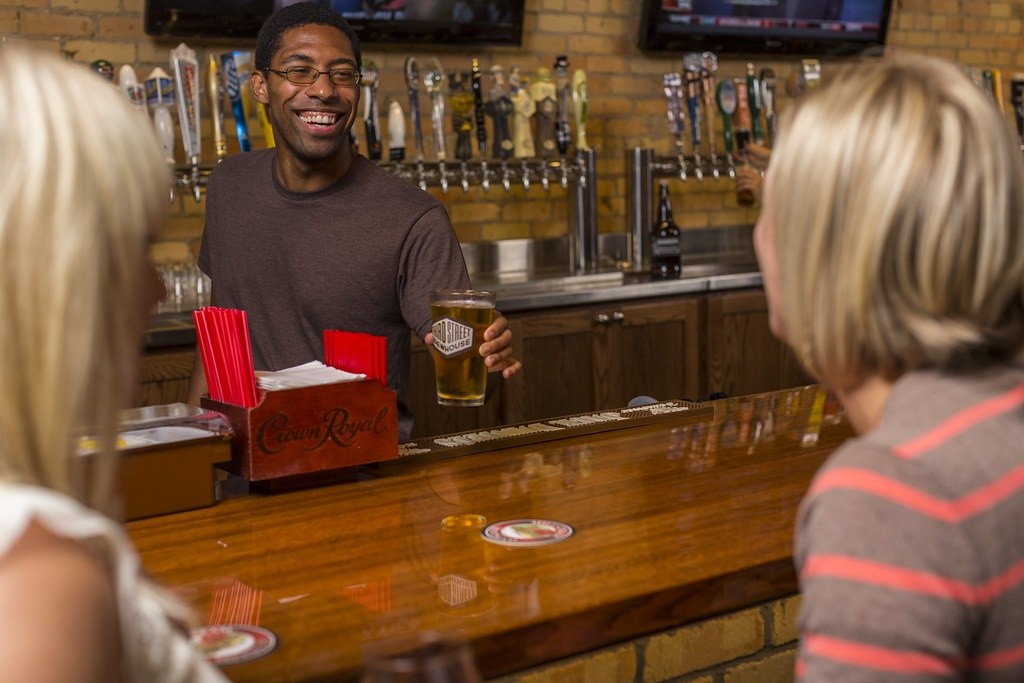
[650,181,683,281]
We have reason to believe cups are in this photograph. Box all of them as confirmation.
[430,288,497,407]
[150,259,212,314]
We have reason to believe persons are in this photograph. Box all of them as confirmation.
[731,143,773,204]
[0,40,242,683]
[751,50,1023,683]
[186,3,522,444]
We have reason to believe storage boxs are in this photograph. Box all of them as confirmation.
[502,284,812,427]
[200,386,400,481]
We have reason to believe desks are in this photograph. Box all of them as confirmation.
[108,378,860,683]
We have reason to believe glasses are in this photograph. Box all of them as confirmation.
[262,65,364,86]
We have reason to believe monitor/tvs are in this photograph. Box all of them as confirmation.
[637,0,892,60]
[143,0,525,45]
